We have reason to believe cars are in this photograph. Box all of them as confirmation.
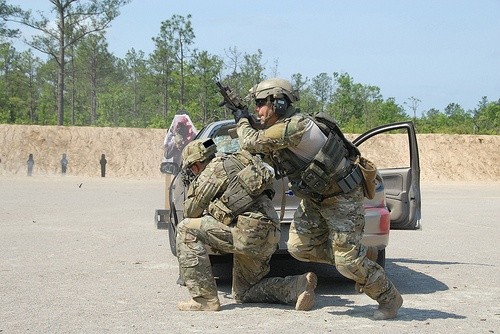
[153,114,423,283]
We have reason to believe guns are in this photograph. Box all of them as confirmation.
[214,81,286,180]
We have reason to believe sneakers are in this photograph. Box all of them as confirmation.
[374,287,403,320]
[355,246,378,294]
[294,272,317,311]
[178,291,221,311]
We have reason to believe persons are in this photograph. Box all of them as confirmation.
[235,79,403,321]
[176,138,318,312]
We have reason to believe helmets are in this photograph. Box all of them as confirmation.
[249,77,294,106]
[181,138,216,170]
